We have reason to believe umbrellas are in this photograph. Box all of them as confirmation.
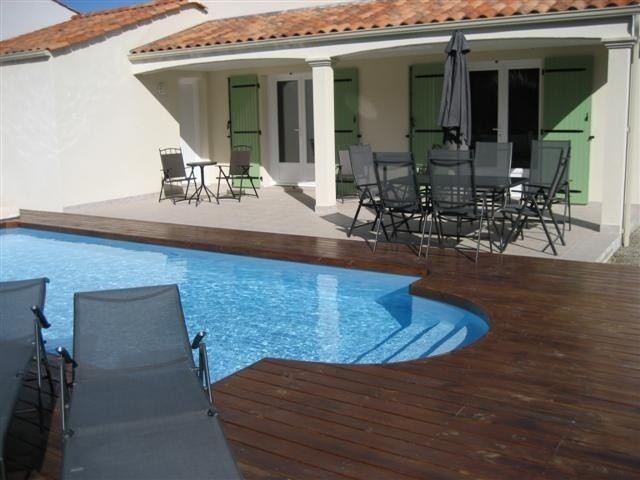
[437,32,471,245]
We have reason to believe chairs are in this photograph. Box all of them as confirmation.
[54,284,244,479]
[214,145,261,205]
[333,137,582,259]
[0,277,52,480]
[157,147,199,206]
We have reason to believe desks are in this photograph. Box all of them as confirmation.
[186,162,220,207]
